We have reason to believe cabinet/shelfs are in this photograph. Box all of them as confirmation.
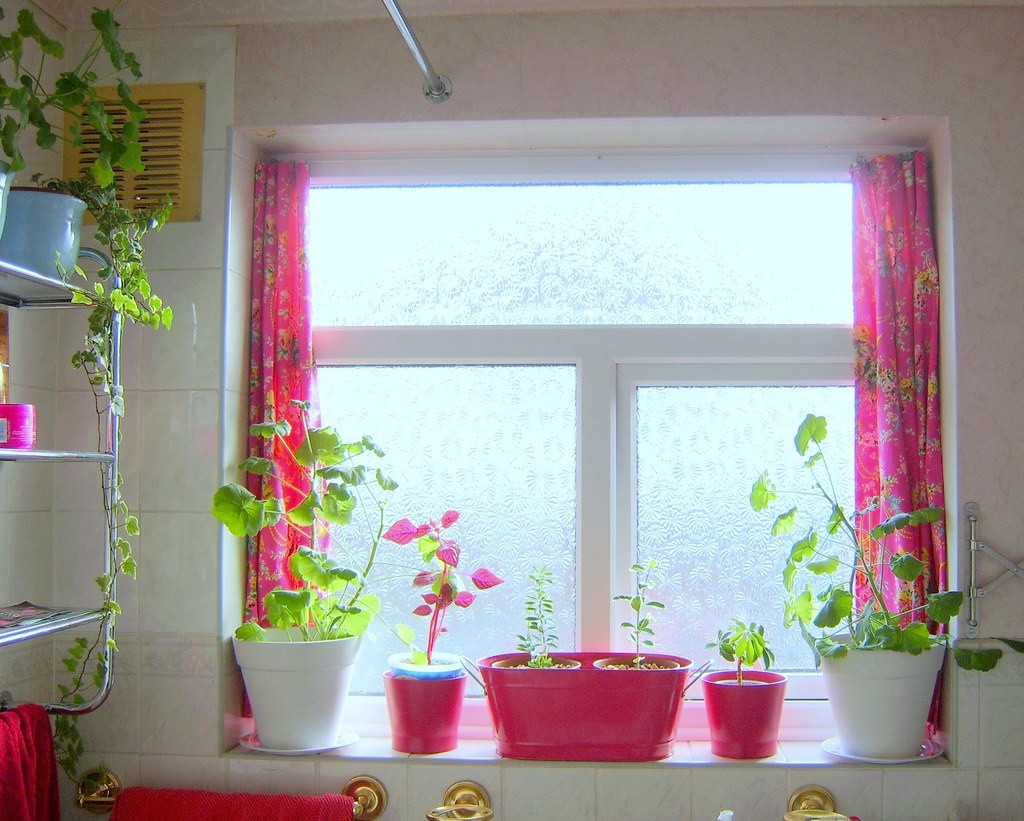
[0,248,126,719]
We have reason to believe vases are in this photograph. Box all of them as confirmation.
[383,671,469,755]
[461,652,713,762]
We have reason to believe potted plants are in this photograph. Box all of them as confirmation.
[382,508,504,682]
[490,564,582,672]
[590,556,680,672]
[0,0,148,236]
[0,171,178,789]
[212,398,400,756]
[747,414,1005,766]
[699,619,791,760]
